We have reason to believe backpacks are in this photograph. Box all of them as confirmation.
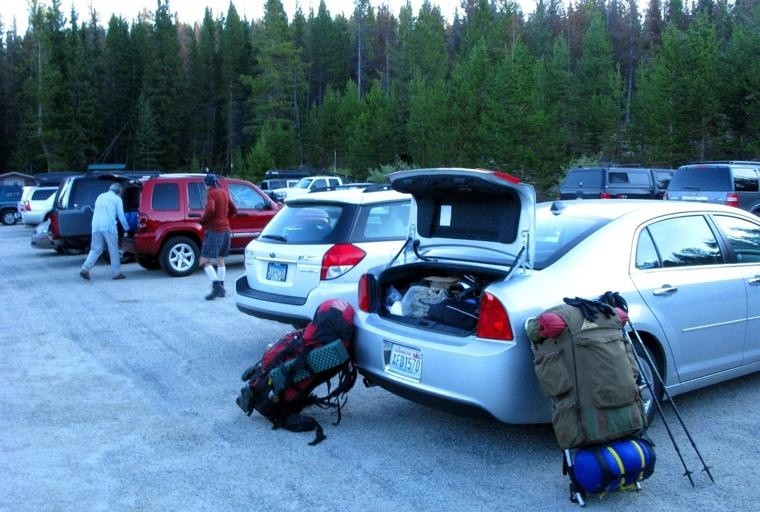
[248,299,354,423]
[528,299,649,447]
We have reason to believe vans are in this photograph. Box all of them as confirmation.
[553,162,681,201]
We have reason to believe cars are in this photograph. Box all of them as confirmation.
[347,164,760,436]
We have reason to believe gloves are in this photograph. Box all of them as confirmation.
[563,297,616,322]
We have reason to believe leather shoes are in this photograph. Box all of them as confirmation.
[206,284,226,299]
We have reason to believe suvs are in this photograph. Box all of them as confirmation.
[229,178,414,334]
[664,158,760,219]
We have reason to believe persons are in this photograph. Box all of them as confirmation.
[79,182,131,281]
[198,173,239,300]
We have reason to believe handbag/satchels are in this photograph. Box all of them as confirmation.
[429,299,479,331]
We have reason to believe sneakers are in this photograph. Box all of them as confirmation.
[79,270,91,280]
[113,273,125,279]
